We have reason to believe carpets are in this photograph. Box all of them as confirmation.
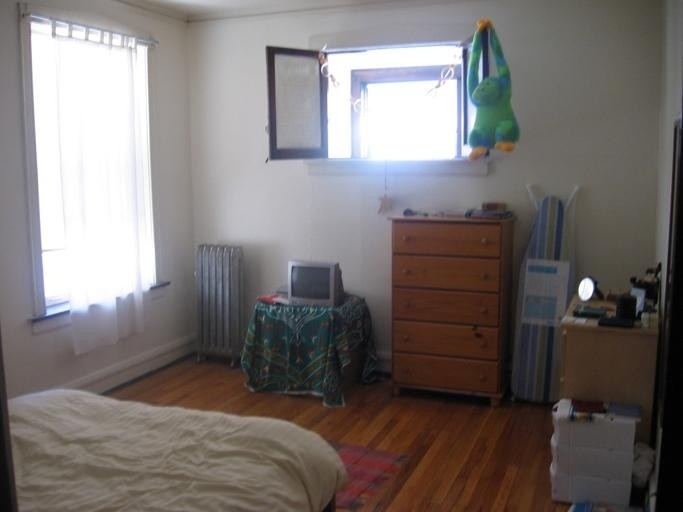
[324,438,424,511]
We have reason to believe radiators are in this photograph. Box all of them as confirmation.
[192,244,246,368]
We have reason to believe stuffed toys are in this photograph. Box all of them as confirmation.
[464,17,520,161]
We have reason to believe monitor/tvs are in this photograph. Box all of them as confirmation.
[288,260,343,305]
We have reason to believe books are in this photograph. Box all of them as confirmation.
[569,397,608,420]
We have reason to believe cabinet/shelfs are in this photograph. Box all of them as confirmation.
[559,289,659,447]
[386,215,518,405]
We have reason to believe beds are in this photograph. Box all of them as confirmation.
[6,387,337,512]
